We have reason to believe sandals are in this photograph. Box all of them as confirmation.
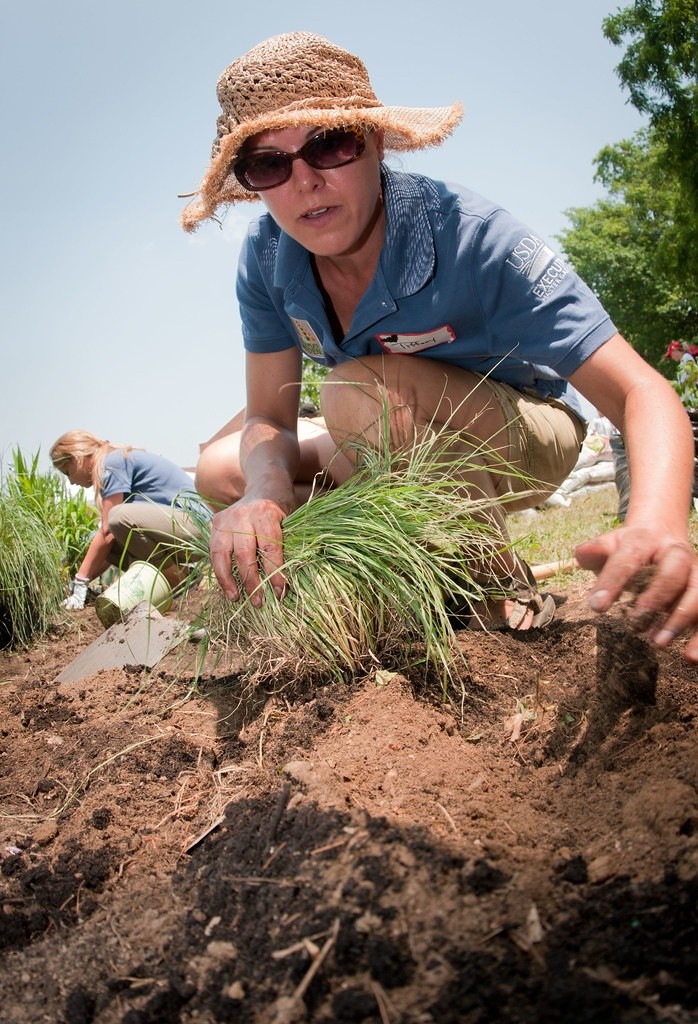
[464,561,556,634]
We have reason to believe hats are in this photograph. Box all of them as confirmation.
[688,344,698,354]
[665,342,682,359]
[177,31,463,235]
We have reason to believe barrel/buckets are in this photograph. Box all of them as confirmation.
[95,560,172,630]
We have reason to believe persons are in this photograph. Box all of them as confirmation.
[176,31,698,668]
[49,430,213,611]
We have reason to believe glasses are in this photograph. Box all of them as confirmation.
[234,124,367,191]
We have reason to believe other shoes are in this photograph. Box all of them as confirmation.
[173,565,207,601]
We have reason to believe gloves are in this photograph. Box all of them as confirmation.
[59,573,92,611]
